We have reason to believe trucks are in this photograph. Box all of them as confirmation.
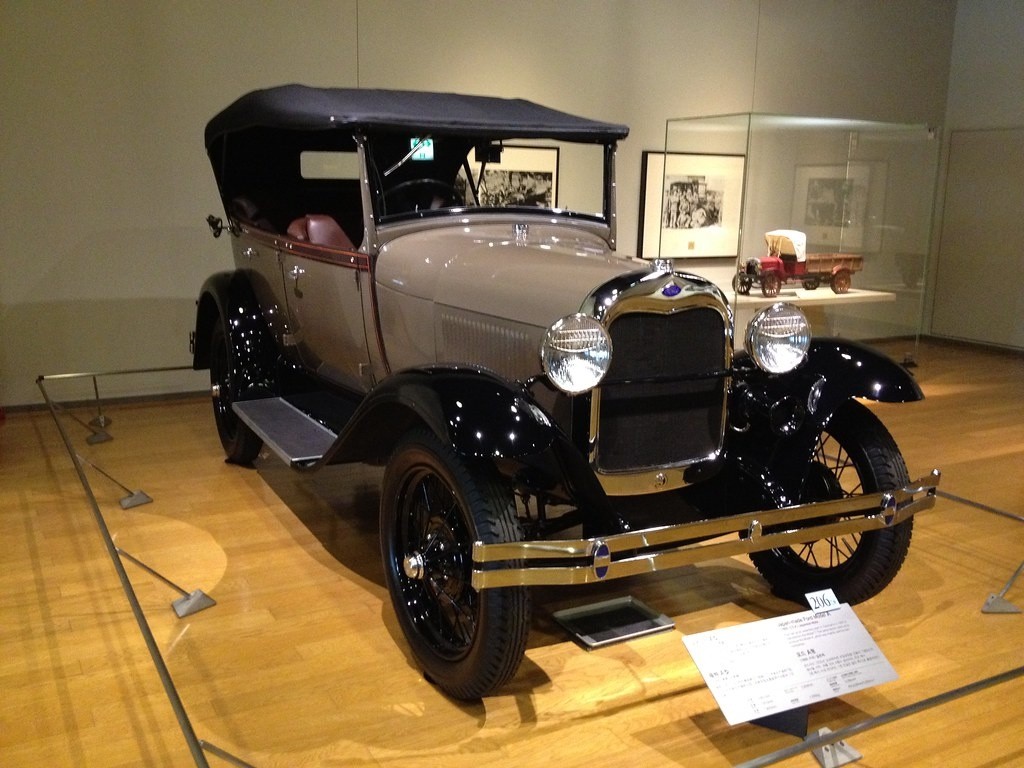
[731,229,865,296]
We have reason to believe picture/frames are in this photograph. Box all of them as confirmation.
[789,162,875,252]
[635,151,746,261]
[457,144,560,208]
[848,156,891,254]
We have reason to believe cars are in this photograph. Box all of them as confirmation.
[188,84,941,701]
